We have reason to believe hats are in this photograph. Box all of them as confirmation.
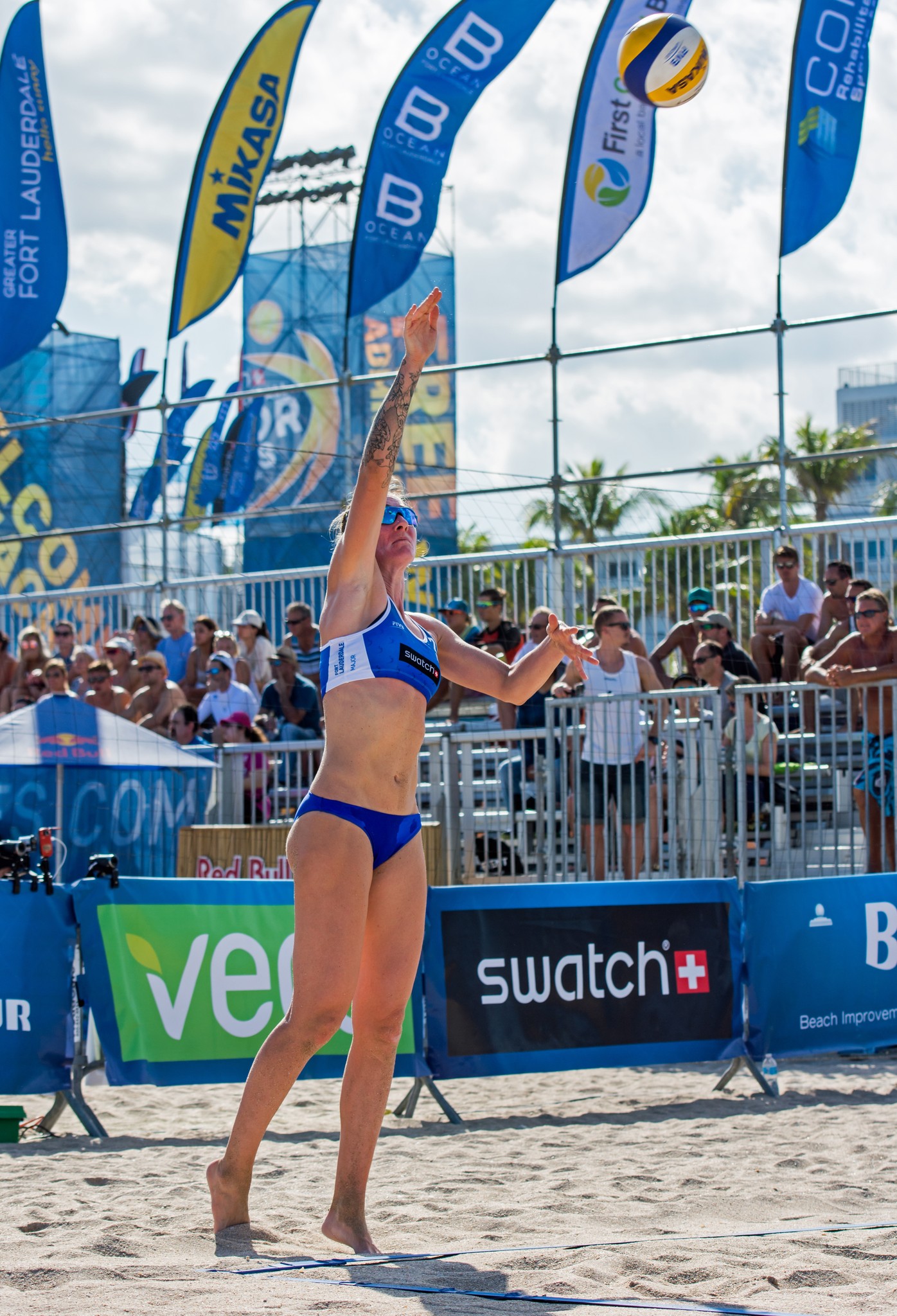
[138,651,170,679]
[209,651,236,680]
[437,597,469,613]
[232,609,263,630]
[16,696,36,705]
[18,626,42,644]
[688,587,714,607]
[105,637,134,655]
[70,644,99,662]
[131,613,162,638]
[220,712,253,730]
[266,646,298,666]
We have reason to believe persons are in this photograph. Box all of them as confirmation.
[205,283,603,1257]
[0,547,897,841]
[550,604,670,881]
[804,588,897,875]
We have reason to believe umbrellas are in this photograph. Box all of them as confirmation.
[0,691,223,883]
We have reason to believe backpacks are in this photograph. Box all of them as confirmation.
[475,837,524,876]
[765,781,801,810]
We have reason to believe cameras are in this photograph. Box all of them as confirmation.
[0,827,55,879]
[86,854,120,878]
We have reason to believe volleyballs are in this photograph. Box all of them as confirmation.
[616,12,709,108]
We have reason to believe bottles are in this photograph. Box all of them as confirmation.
[763,1053,778,1095]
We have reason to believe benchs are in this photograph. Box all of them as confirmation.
[279,695,879,883]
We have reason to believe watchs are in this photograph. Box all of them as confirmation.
[648,735,659,746]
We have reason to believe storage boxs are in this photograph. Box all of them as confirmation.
[0,1106,27,1143]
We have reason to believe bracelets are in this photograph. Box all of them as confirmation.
[769,617,775,624]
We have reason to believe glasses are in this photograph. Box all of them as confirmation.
[223,724,243,728]
[206,668,220,675]
[608,622,631,631]
[825,578,838,586]
[21,640,39,649]
[285,617,306,625]
[30,682,47,690]
[844,595,856,603]
[476,601,501,608]
[55,629,72,637]
[88,676,105,684]
[590,610,596,616]
[382,505,419,527]
[137,665,162,673]
[700,624,719,630]
[161,615,174,621]
[529,623,542,630]
[855,609,883,618]
[690,604,711,612]
[44,672,59,679]
[135,625,148,632]
[441,610,465,615]
[690,654,716,664]
[214,629,232,639]
[725,700,736,708]
[776,560,797,569]
[270,660,282,667]
[104,649,121,655]
